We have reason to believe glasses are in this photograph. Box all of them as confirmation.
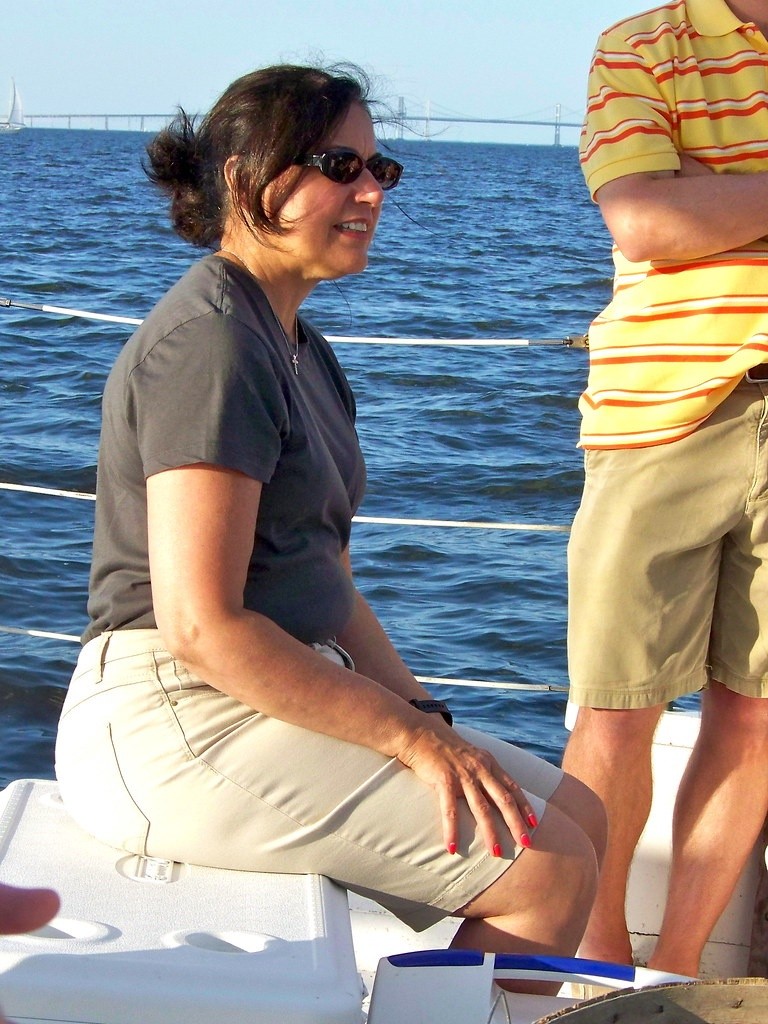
[292,147,404,190]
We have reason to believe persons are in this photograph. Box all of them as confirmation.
[560,0,768,1002]
[54,64,609,998]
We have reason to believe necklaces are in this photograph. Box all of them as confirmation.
[219,247,300,377]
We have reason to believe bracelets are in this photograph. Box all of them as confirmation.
[408,697,455,728]
